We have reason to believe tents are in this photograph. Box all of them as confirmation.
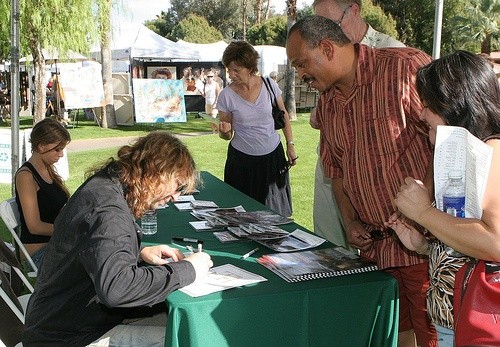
[88,23,288,130]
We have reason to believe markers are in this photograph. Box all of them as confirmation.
[171,236,204,244]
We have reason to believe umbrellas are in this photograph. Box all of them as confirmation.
[19,43,88,113]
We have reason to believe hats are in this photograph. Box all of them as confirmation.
[207,73,213,77]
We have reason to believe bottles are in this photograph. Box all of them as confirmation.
[443,171,466,257]
[140,209,157,236]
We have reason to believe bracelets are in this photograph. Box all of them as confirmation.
[416,239,433,255]
[287,141,294,145]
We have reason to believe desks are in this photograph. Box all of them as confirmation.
[136,170,399,347]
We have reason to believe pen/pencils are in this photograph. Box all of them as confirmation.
[197,243,203,254]
[239,246,260,261]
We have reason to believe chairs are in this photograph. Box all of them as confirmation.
[0,197,38,274]
[0,241,34,325]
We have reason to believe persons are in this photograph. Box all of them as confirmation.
[203,71,220,133]
[312,0,408,252]
[285,15,440,346]
[217,41,298,218]
[22,134,213,347]
[384,53,500,347]
[30,74,66,119]
[13,118,73,274]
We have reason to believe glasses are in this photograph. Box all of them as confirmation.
[176,179,188,191]
[365,227,393,241]
[334,5,353,26]
[278,156,299,177]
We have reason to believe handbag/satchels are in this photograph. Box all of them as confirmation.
[453,257,500,347]
[261,75,286,130]
